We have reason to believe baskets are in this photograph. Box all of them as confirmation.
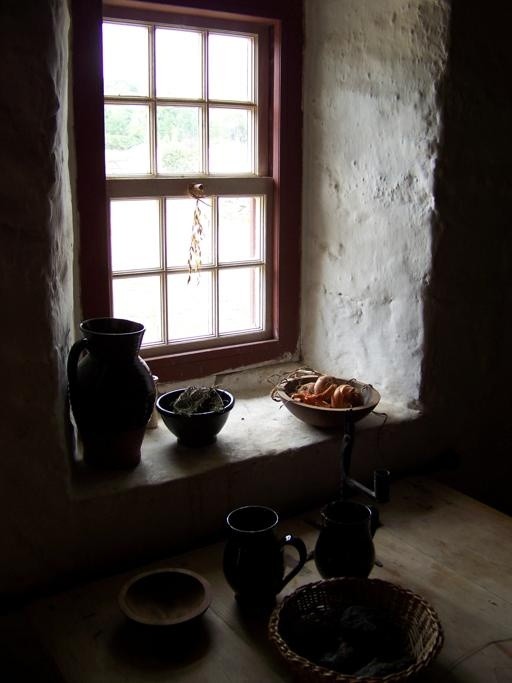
[268,576,444,683]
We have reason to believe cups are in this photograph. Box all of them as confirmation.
[314,500,380,581]
[221,505,308,603]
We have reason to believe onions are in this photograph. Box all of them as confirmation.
[297,375,360,408]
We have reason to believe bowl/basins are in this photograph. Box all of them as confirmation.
[155,386,236,447]
[117,566,213,630]
[276,374,381,429]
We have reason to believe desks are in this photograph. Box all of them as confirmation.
[29,472,512,682]
